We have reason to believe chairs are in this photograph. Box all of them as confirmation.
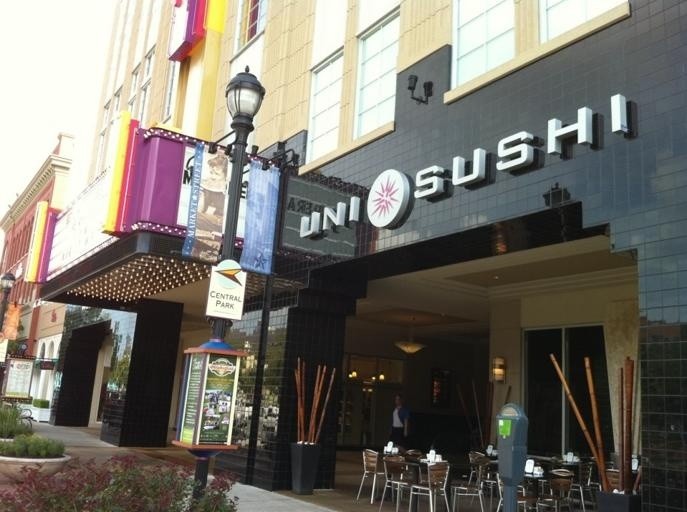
[360,442,615,512]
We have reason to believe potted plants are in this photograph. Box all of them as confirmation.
[0,401,71,484]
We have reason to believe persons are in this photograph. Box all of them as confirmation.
[197,149,230,233]
[392,391,416,448]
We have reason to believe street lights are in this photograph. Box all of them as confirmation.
[170,65,266,511]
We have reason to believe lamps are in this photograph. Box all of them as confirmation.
[393,326,429,356]
[488,356,507,385]
